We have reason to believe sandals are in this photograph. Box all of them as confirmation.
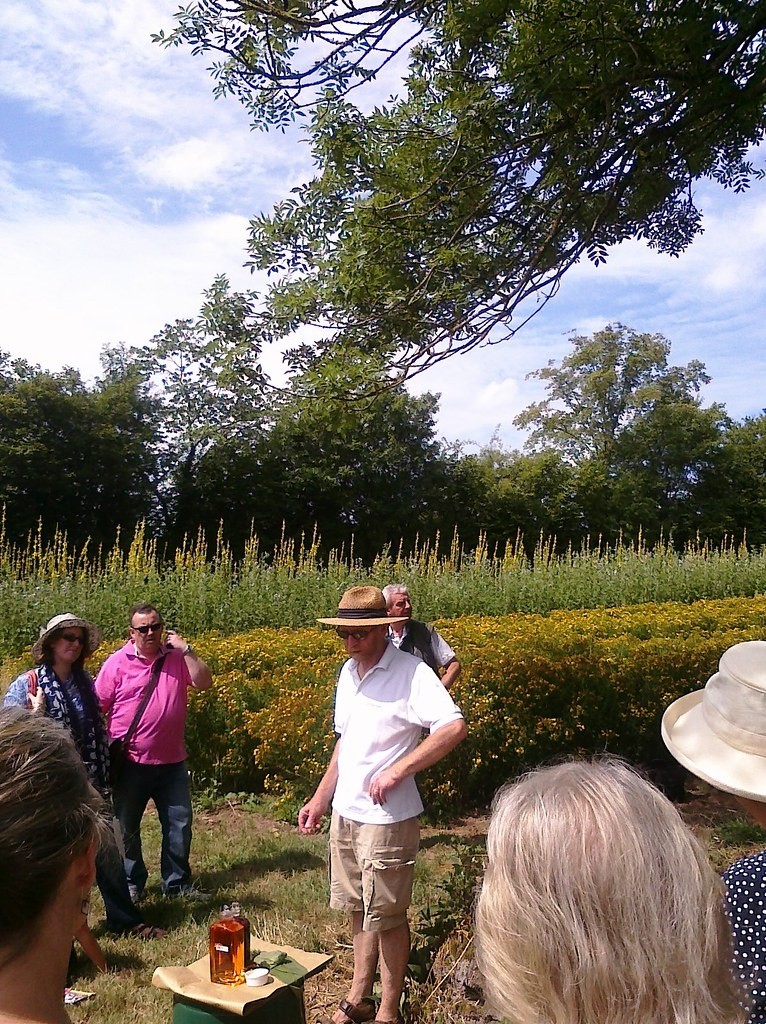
[121,922,169,940]
[370,1009,406,1024]
[317,997,379,1024]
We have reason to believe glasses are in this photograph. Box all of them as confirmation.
[335,625,378,641]
[61,633,88,644]
[131,622,165,633]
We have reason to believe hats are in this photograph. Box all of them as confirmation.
[316,586,410,626]
[661,641,766,803]
[32,613,102,664]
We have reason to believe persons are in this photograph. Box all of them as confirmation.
[298,587,467,1024]
[94,605,213,901]
[473,756,751,1024]
[661,641,766,1024]
[0,706,119,1024]
[382,584,461,690]
[4,612,168,942]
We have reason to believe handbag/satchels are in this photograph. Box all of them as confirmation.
[110,738,130,787]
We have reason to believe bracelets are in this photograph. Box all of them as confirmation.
[183,645,191,656]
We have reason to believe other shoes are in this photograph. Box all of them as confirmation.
[129,883,144,904]
[163,887,213,902]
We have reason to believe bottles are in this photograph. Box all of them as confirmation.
[209,901,251,988]
